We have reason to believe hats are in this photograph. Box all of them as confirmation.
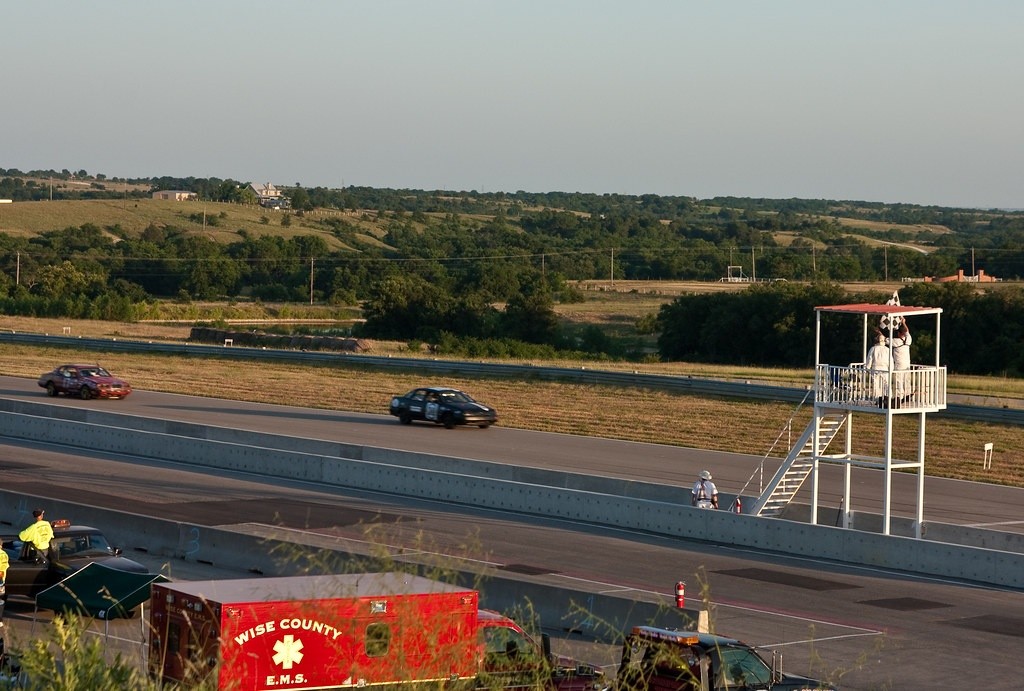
[874,335,885,342]
[32,510,45,518]
[698,470,713,480]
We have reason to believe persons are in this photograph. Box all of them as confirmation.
[690,468,720,511]
[865,331,895,408]
[881,316,913,403]
[19,506,55,557]
[0,538,10,629]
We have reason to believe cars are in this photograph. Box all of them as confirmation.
[38,364,131,399]
[2,519,153,612]
[619,627,836,691]
[386,386,500,430]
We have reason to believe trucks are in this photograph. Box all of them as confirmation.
[146,571,605,691]
